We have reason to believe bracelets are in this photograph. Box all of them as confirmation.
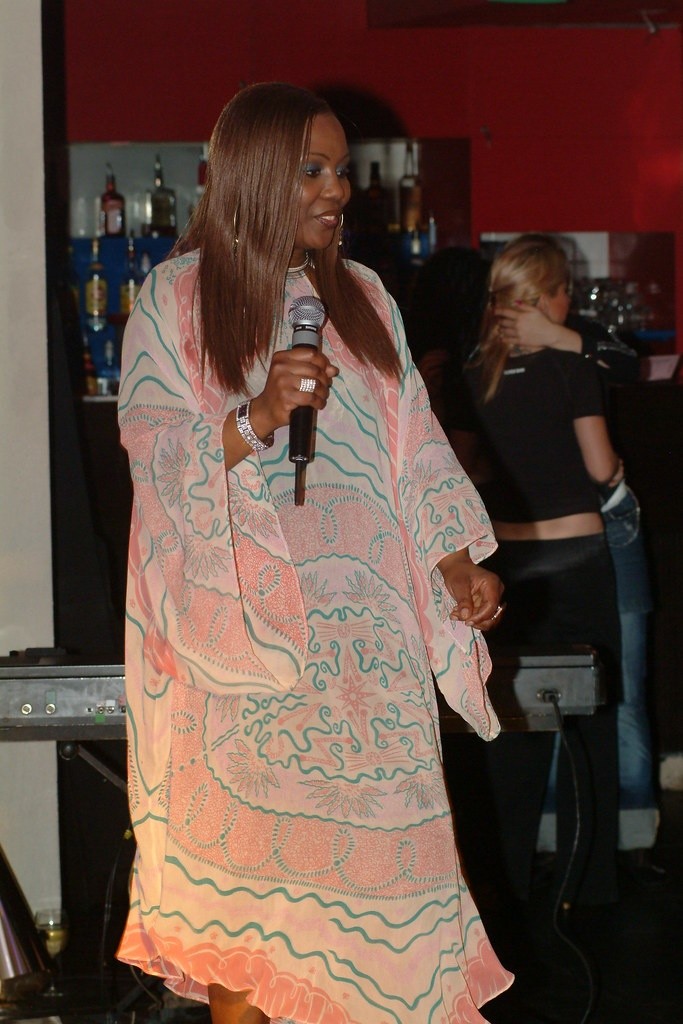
[236,399,274,453]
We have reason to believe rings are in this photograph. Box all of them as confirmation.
[298,378,316,393]
[491,606,504,623]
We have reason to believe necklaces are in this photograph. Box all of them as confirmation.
[287,251,309,272]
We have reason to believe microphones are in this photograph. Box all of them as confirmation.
[288,295,325,507]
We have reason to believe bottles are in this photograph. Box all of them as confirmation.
[77,138,435,399]
[568,278,664,340]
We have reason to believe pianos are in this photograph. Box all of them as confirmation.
[0,644,609,743]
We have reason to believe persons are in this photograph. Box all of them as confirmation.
[404,235,658,959]
[115,82,517,1024]
